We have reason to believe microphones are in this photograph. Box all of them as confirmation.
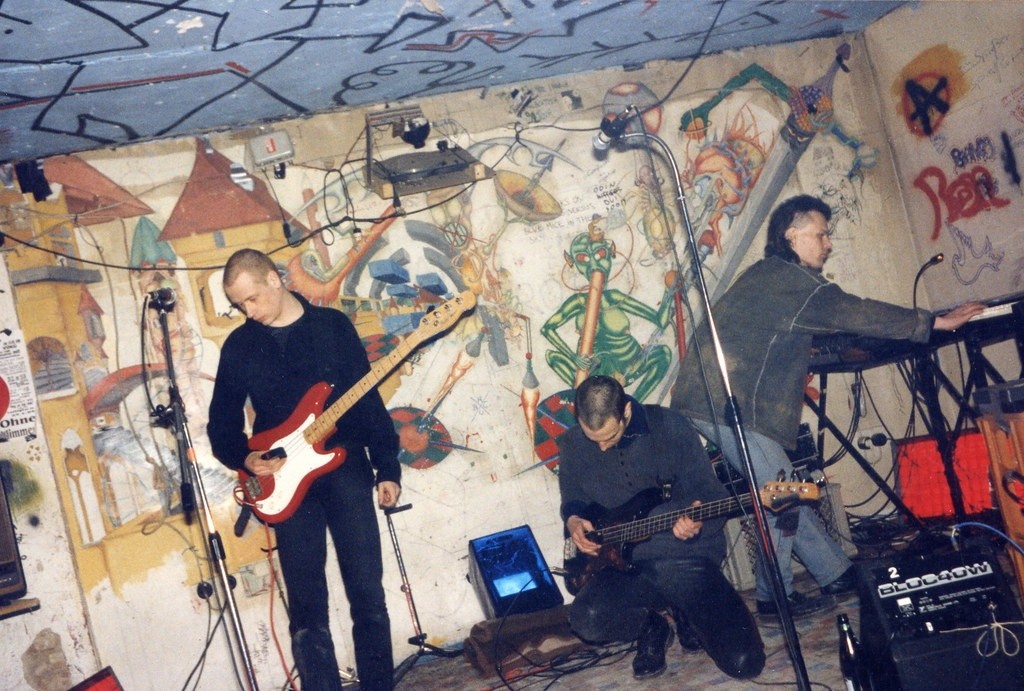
[146,286,177,306]
[594,105,633,151]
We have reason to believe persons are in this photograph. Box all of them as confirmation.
[669,192,989,623]
[206,248,402,691]
[557,374,767,681]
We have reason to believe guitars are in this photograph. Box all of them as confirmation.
[561,467,827,596]
[237,289,476,525]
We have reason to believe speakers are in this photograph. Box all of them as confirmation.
[467,524,564,620]
[854,540,1024,691]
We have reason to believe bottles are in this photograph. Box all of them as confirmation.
[836,613,874,691]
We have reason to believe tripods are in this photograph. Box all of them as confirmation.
[384,504,463,688]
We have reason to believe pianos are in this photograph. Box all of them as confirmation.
[809,291,1024,373]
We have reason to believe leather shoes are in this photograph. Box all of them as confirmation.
[672,609,703,651]
[633,613,675,680]
[820,576,858,603]
[756,590,837,620]
